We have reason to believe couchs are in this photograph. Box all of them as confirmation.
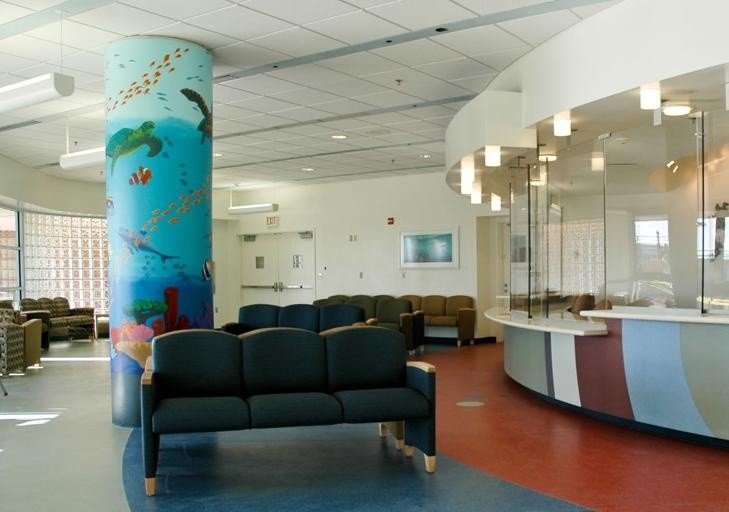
[0,290,112,394]
[137,323,439,494]
[401,291,476,348]
[214,302,376,335]
[313,294,423,351]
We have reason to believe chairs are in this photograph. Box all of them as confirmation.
[592,300,612,310]
[569,291,596,313]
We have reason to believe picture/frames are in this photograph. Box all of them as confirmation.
[400,226,459,269]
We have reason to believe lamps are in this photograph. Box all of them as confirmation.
[58,124,106,168]
[461,80,681,214]
[0,8,75,113]
[228,190,279,215]
